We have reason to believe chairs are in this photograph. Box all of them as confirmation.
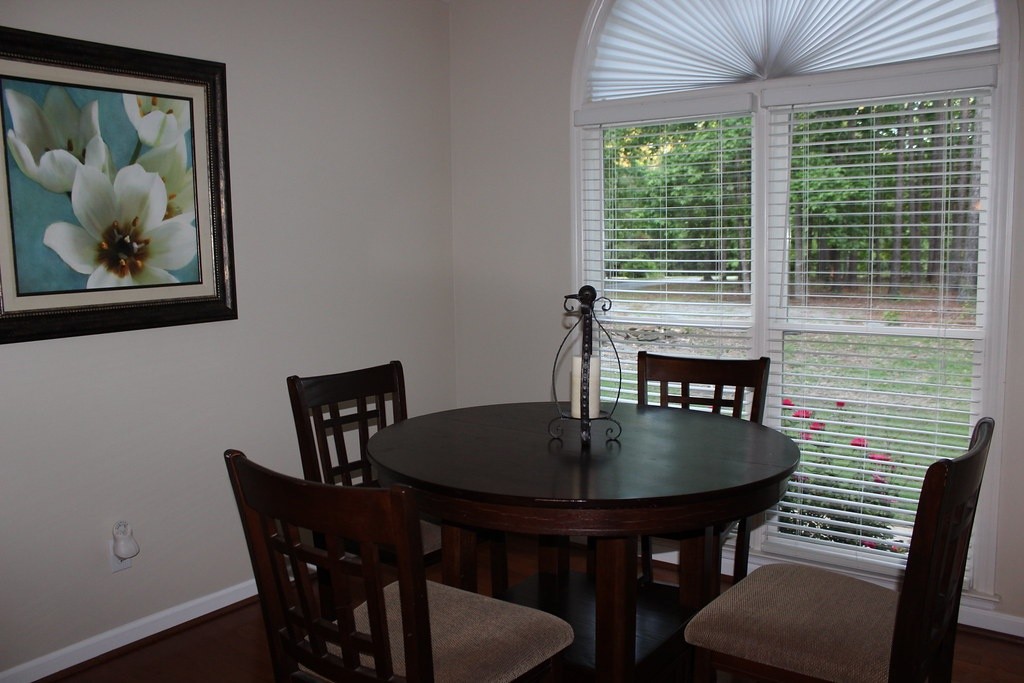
[219,452,576,682]
[683,416,994,683]
[635,348,771,615]
[283,359,508,612]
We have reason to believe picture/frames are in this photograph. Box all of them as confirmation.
[0,26,239,345]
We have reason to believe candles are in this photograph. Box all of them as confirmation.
[572,354,600,418]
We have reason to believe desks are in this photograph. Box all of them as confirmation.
[362,402,799,683]
